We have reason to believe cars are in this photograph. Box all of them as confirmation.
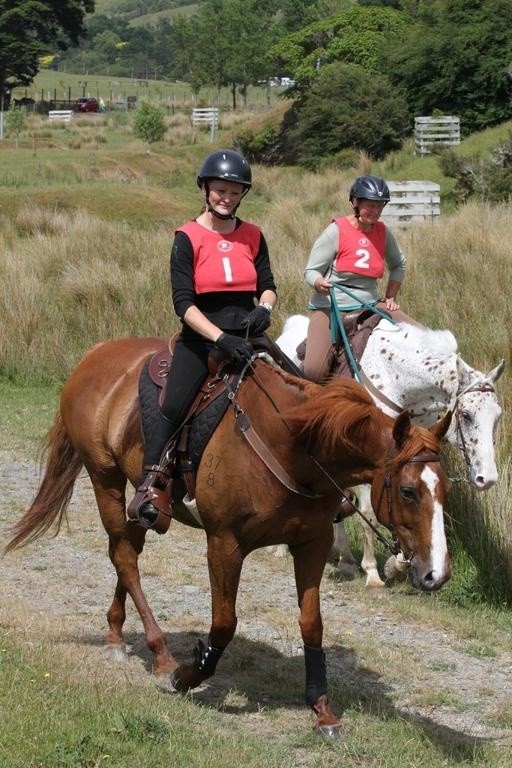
[73,95,100,113]
[257,75,302,86]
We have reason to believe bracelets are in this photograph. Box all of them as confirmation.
[258,302,272,312]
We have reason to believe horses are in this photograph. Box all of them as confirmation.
[0,336,452,753]
[274,314,506,589]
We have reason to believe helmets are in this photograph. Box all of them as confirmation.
[196,149,254,189]
[347,174,393,204]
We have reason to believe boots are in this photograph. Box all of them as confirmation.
[138,408,182,531]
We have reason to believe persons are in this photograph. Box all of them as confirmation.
[127,149,278,533]
[302,174,422,515]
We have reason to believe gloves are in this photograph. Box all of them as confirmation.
[239,305,275,336]
[216,330,255,368]
